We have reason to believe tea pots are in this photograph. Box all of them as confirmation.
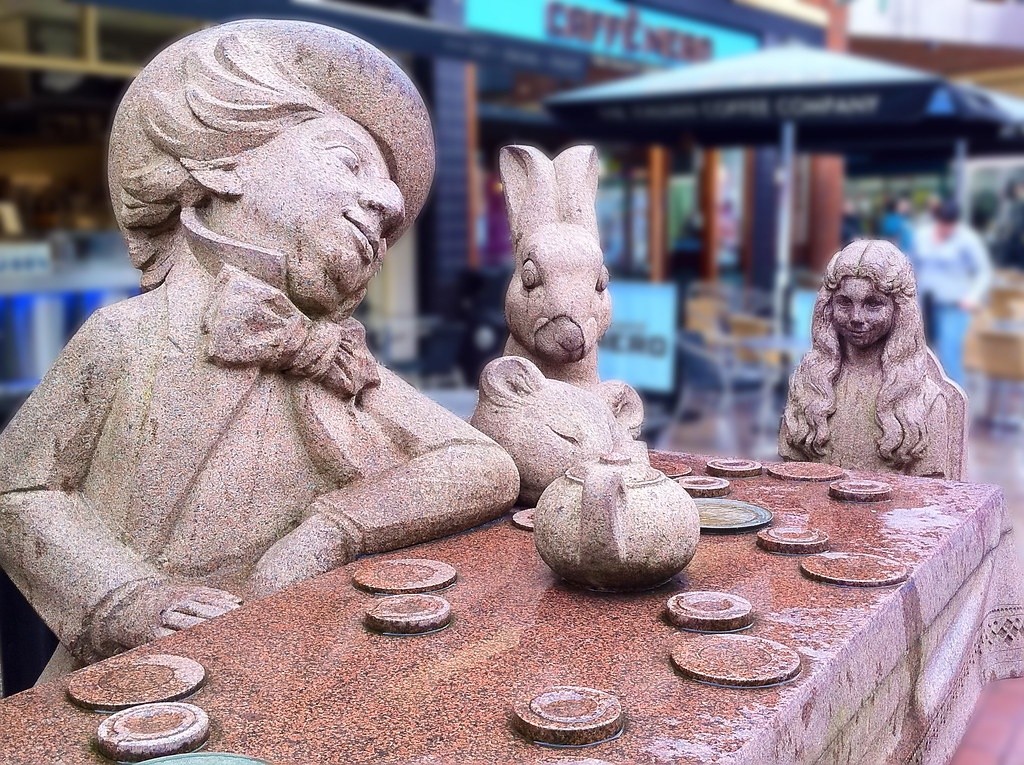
[530,439,701,592]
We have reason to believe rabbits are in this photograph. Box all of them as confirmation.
[498,144,610,393]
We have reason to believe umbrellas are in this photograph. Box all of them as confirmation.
[537,38,1024,451]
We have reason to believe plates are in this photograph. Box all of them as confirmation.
[129,753,266,765]
[688,495,772,530]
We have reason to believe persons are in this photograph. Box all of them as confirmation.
[840,199,862,249]
[880,195,914,252]
[779,239,949,483]
[1,17,519,689]
[990,176,1024,273]
[915,198,992,390]
